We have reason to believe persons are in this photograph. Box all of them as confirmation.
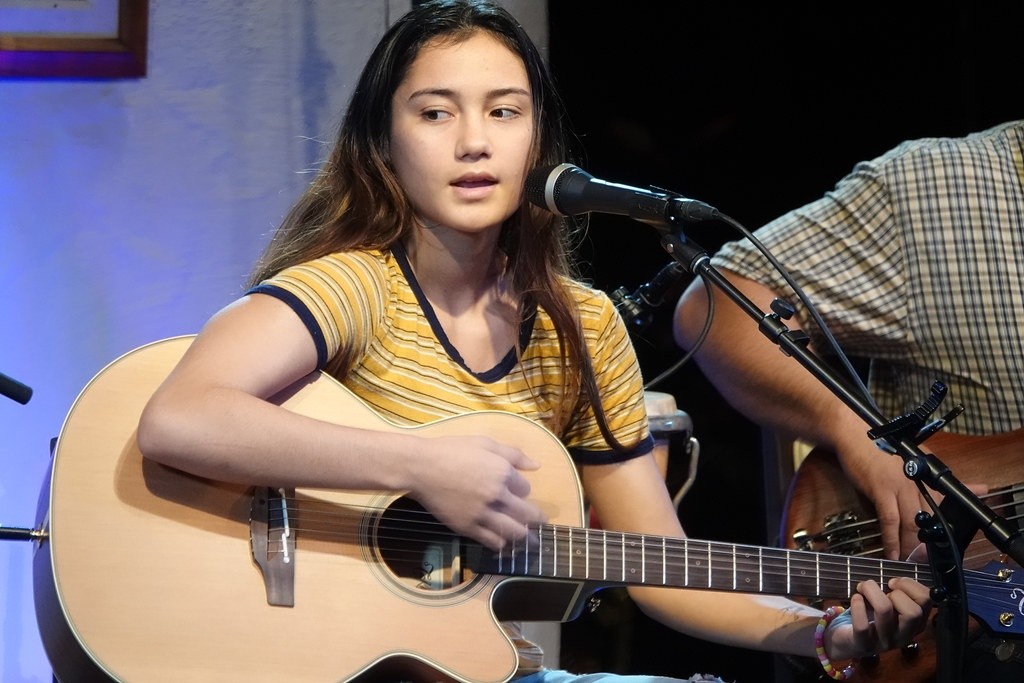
[140,0,941,683]
[674,119,1024,564]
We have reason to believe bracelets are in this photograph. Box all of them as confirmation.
[814,604,863,681]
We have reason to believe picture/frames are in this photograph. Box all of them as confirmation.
[0,0,147,78]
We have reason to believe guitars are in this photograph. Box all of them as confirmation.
[29,331,1024,683]
[776,414,1024,683]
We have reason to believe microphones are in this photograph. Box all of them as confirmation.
[527,161,721,226]
